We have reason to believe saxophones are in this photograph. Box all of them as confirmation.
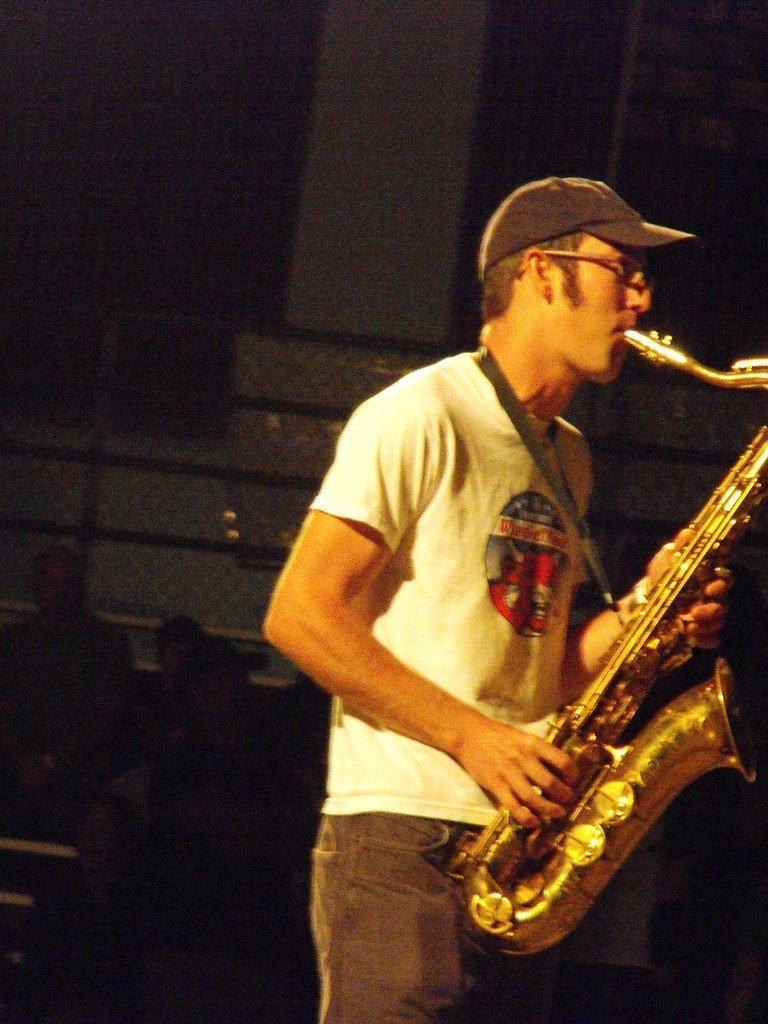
[443,324,767,961]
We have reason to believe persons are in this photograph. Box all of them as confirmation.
[258,177,730,1024]
[0,549,768,1024]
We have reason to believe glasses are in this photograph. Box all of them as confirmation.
[517,250,664,296]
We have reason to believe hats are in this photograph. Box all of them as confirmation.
[480,177,700,272]
